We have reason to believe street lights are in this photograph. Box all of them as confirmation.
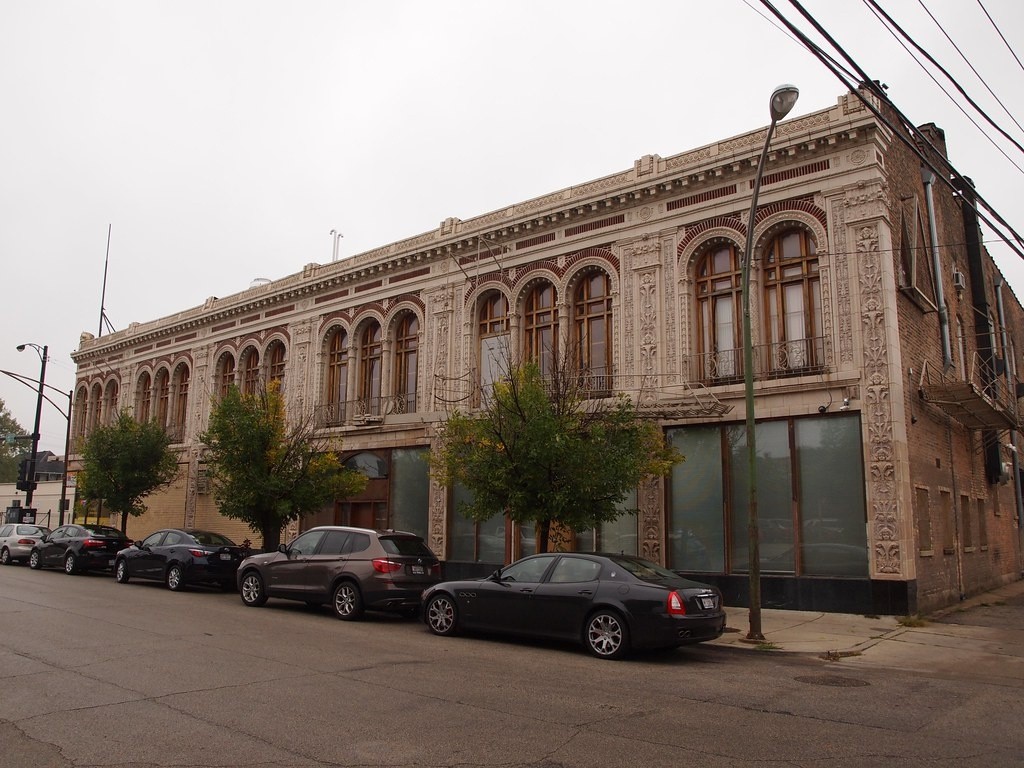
[737,84,802,646]
[16,342,51,508]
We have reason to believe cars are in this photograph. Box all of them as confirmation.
[28,523,133,574]
[112,528,250,592]
[0,524,55,565]
[422,550,729,658]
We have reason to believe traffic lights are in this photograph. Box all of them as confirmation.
[17,459,27,482]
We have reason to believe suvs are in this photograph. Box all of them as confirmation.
[234,525,444,620]
